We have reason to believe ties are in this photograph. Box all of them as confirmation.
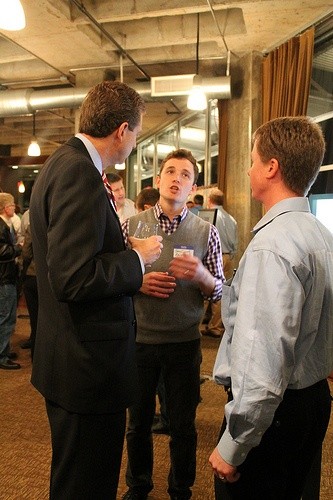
[102,171,118,211]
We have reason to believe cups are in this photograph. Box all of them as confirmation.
[17,236,24,246]
[134,221,158,239]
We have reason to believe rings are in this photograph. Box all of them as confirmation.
[219,476,225,479]
[184,269,189,274]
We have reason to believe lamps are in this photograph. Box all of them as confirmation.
[27,113,41,156]
[186,12,208,110]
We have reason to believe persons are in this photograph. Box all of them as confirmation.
[106,170,136,224]
[30,82,163,500]
[134,187,169,433]
[209,117,333,500]
[120,148,227,500]
[186,186,238,337]
[0,192,37,369]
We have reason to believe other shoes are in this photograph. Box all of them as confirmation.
[200,329,221,338]
[151,423,168,435]
[121,492,148,500]
[169,494,191,500]
[20,343,30,348]
[4,352,17,359]
[0,360,20,370]
